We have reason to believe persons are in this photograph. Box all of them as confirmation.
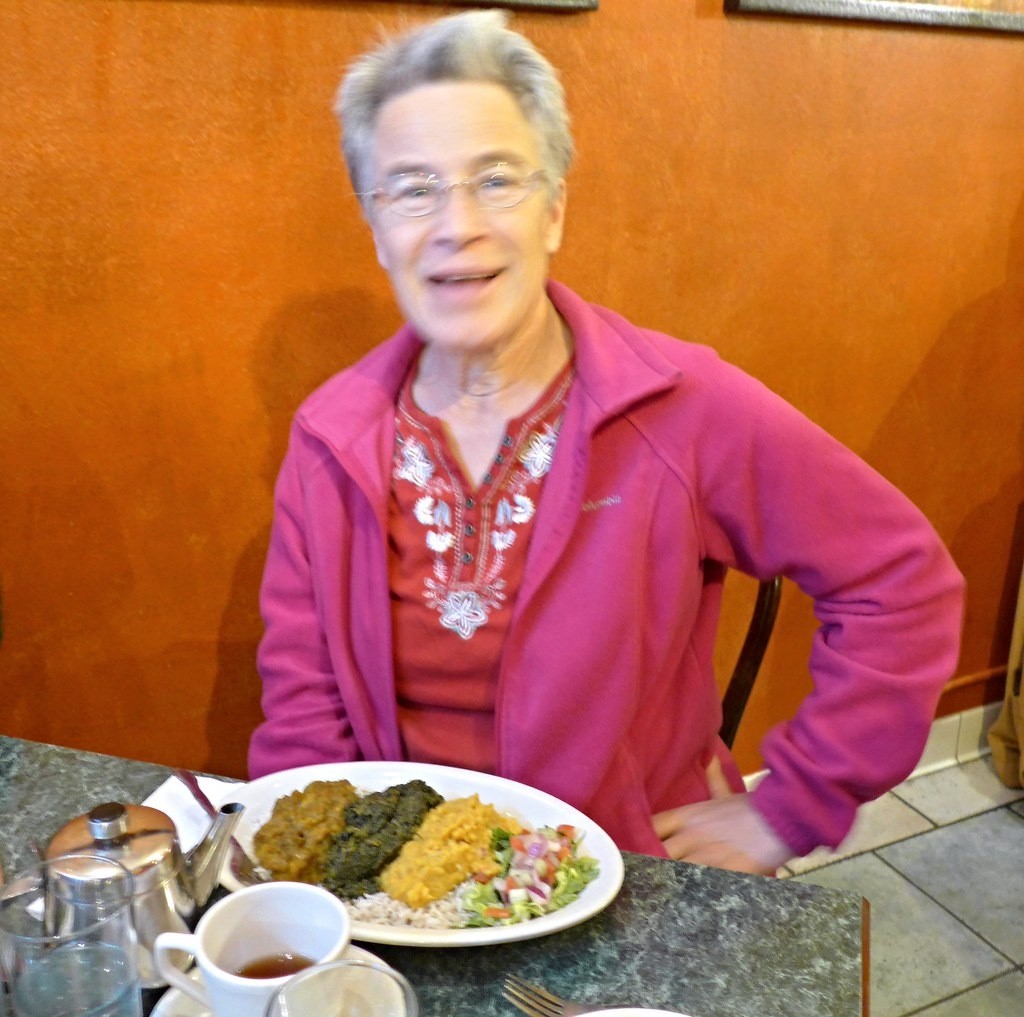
[248,6,966,879]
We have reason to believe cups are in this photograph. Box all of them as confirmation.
[264,959,423,1017]
[0,852,145,1017]
[152,881,357,1017]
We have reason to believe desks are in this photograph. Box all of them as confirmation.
[0,735,870,1017]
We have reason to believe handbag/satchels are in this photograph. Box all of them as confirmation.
[986,696,1024,789]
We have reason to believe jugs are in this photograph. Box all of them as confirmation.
[0,798,249,994]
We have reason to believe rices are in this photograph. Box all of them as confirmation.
[348,880,478,927]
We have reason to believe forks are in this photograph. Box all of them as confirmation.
[501,973,681,1017]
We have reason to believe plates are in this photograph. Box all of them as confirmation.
[148,944,395,1017]
[202,760,625,949]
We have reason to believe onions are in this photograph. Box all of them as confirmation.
[492,829,560,903]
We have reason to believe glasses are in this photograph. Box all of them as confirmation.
[358,162,553,218]
